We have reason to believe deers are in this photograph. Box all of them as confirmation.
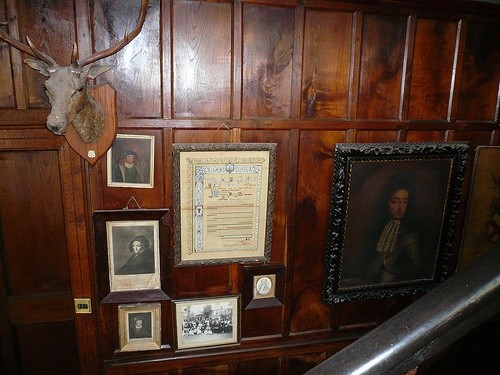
[0,0,149,144]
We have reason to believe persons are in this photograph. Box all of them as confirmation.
[183,311,232,335]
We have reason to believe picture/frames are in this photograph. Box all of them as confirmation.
[172,141,277,269]
[171,294,241,353]
[105,220,163,294]
[117,303,161,352]
[106,134,155,189]
[320,142,469,307]
[455,145,500,275]
